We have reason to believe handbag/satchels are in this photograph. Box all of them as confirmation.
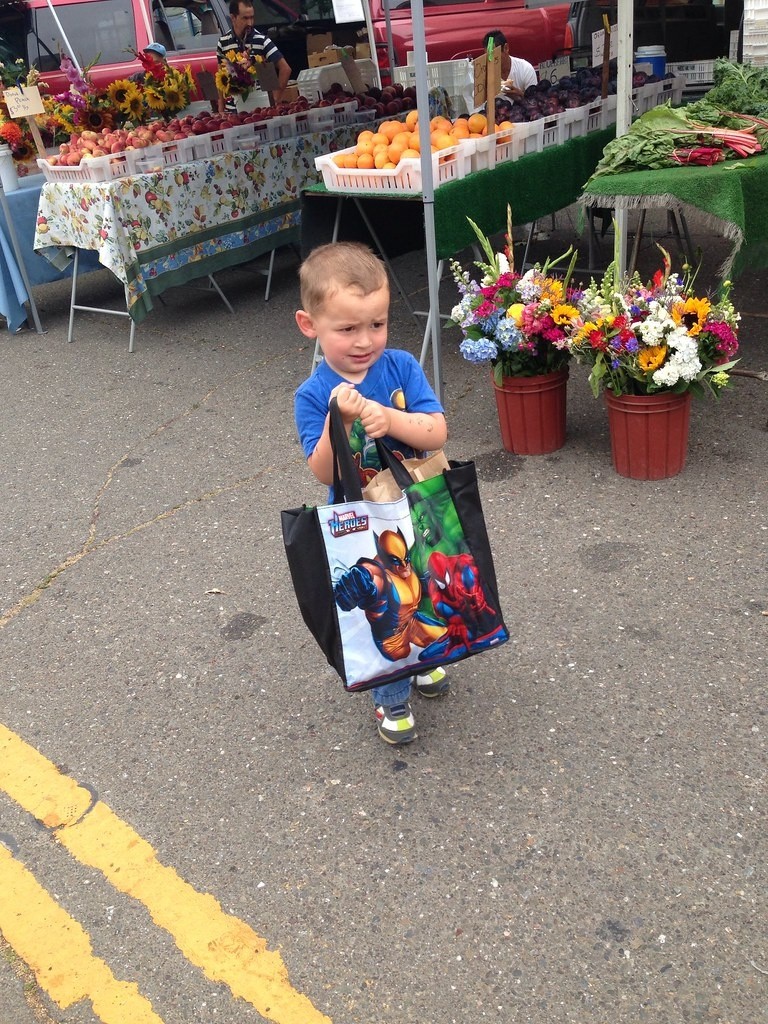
[280,397,510,693]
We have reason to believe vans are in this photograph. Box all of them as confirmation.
[564,0,745,105]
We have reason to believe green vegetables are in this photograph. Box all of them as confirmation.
[580,57,768,189]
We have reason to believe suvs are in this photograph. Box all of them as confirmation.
[1,0,305,129]
[368,0,572,91]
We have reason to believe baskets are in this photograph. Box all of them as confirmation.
[36,100,377,183]
[297,58,379,104]
[664,60,716,85]
[393,58,472,112]
[314,71,686,195]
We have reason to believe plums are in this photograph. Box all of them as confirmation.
[459,67,659,122]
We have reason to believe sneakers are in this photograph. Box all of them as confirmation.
[375,701,418,744]
[410,666,450,697]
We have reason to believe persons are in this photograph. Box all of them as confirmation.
[480,29,538,100]
[295,242,449,744]
[127,43,176,111]
[216,1,290,114]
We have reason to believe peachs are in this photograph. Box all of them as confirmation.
[46,119,188,170]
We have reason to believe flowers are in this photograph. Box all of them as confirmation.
[0,63,35,164]
[581,243,740,403]
[215,49,262,101]
[447,203,590,377]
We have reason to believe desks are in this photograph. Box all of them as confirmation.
[33,108,434,351]
[580,151,768,319]
[300,102,689,377]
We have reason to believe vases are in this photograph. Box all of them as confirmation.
[489,362,570,455]
[603,382,691,481]
[0,144,19,194]
[236,91,271,113]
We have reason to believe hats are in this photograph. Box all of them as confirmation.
[143,43,166,57]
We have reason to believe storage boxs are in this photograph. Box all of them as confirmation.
[394,57,470,116]
[274,31,380,102]
[37,101,359,183]
[665,59,716,84]
[314,77,682,194]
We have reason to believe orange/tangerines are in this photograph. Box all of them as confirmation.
[333,109,513,171]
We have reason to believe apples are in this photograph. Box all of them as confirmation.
[183,82,416,145]
[36,127,360,276]
